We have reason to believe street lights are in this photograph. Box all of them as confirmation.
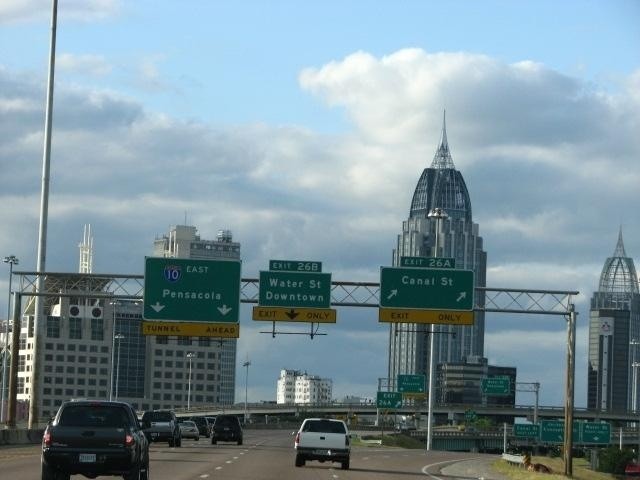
[243,361,251,423]
[110,301,120,402]
[186,352,195,410]
[423,205,450,450]
[0,255,21,425]
[114,332,125,401]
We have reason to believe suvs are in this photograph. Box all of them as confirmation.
[38,398,149,480]
[291,417,352,469]
[141,410,183,448]
[211,415,243,446]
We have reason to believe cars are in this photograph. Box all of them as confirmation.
[179,421,200,441]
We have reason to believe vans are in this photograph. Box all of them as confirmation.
[191,416,210,438]
[205,416,216,432]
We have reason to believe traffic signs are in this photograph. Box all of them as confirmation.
[401,256,456,269]
[379,310,474,326]
[141,256,241,325]
[143,320,241,340]
[379,266,475,310]
[270,261,322,272]
[374,371,612,446]
[251,305,336,323]
[257,270,330,307]
